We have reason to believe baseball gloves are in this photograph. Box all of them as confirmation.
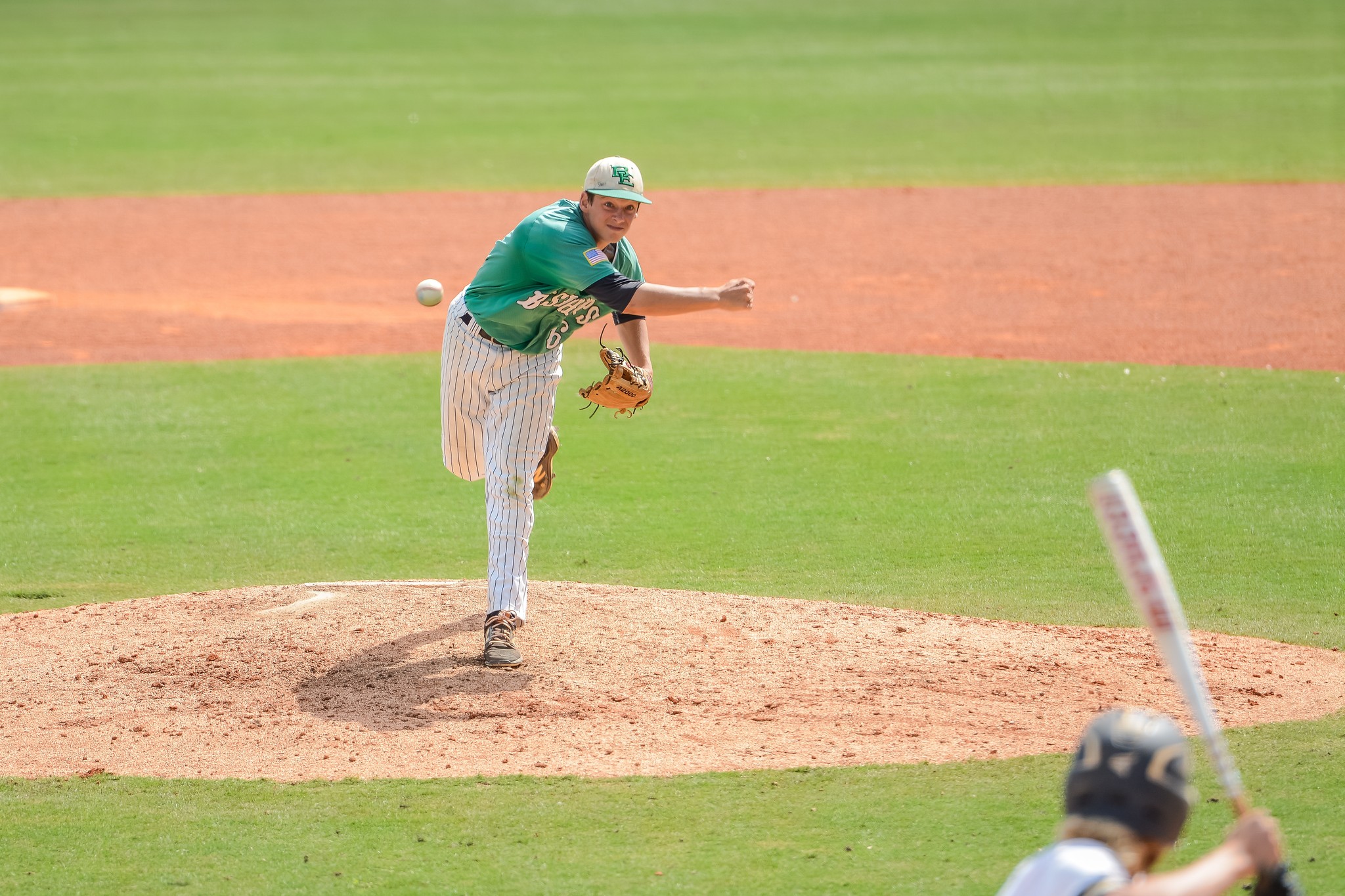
[578,348,652,409]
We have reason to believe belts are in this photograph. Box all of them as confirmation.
[459,312,506,346]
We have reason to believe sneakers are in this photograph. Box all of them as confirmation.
[484,610,523,666]
[531,425,562,500]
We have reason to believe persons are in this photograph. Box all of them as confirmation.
[437,154,757,669]
[991,707,1285,896]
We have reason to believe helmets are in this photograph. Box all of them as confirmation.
[1063,707,1197,847]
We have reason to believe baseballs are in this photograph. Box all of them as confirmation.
[416,279,444,306]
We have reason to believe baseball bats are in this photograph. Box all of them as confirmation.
[1088,467,1301,896]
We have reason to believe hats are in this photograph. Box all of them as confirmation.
[584,155,652,205]
